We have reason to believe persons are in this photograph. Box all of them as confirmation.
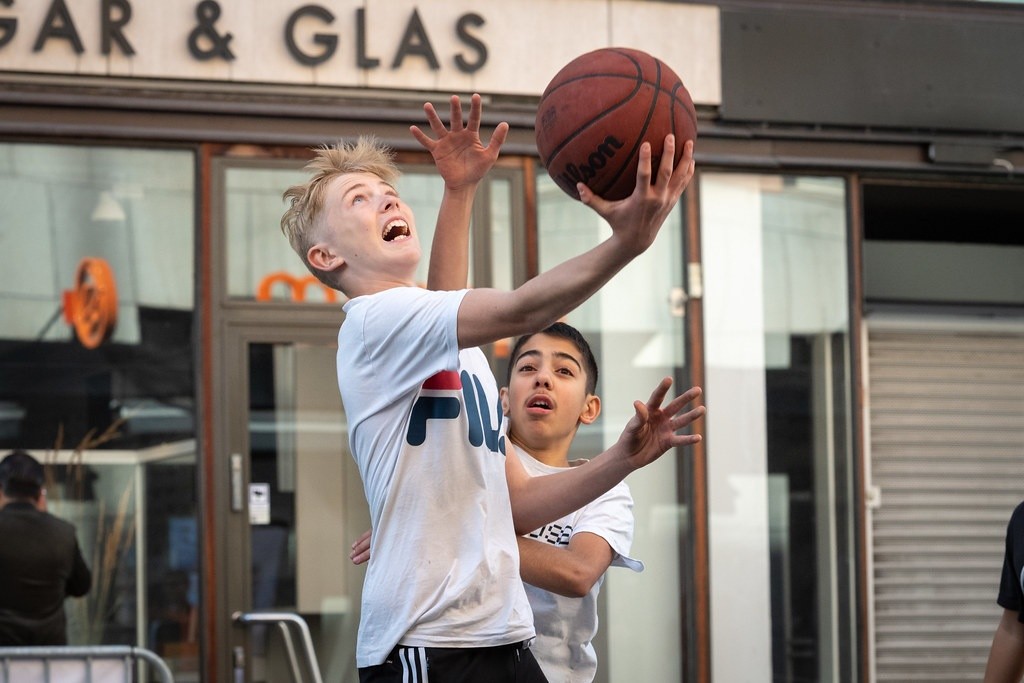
[984,501,1024,683]
[280,94,707,683]
[1,451,93,646]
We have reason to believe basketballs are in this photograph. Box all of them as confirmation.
[534,46,697,203]
